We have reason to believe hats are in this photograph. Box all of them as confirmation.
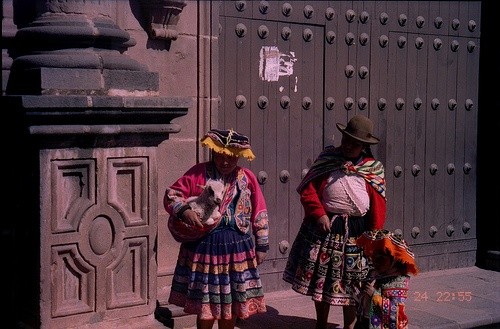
[200,128,255,162]
[336,116,380,144]
[354,229,417,275]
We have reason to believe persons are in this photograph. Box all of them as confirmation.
[163,127,270,329]
[356,229,420,328]
[283,115,387,329]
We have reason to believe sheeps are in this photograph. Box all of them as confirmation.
[184,177,227,225]
